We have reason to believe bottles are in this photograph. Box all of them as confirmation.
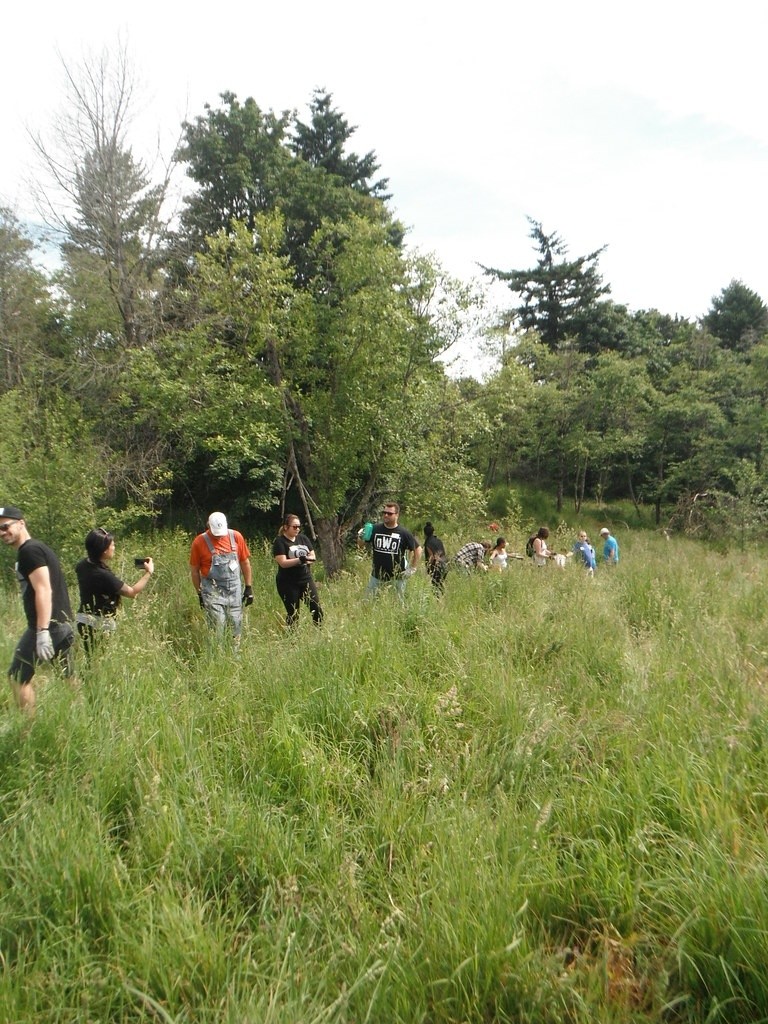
[361,520,373,541]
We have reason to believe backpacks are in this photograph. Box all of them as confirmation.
[526,537,542,557]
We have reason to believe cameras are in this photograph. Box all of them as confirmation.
[135,559,149,569]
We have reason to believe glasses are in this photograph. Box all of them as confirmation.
[0,521,19,531]
[288,525,300,530]
[381,511,397,516]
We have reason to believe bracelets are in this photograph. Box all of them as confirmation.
[37,626,49,633]
[147,571,151,575]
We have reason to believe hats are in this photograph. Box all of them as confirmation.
[0,506,25,520]
[208,512,228,537]
[599,528,610,536]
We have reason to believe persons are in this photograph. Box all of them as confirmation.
[190,512,254,643]
[454,542,491,575]
[533,527,557,567]
[75,527,154,658]
[565,531,596,577]
[489,537,507,568]
[273,514,323,625]
[358,502,422,599]
[599,528,619,567]
[424,522,449,599]
[0,506,85,715]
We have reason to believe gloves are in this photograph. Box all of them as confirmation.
[299,556,316,565]
[198,592,205,609]
[400,569,416,580]
[549,556,556,561]
[357,528,366,539]
[36,630,55,661]
[242,585,254,607]
[550,553,557,556]
